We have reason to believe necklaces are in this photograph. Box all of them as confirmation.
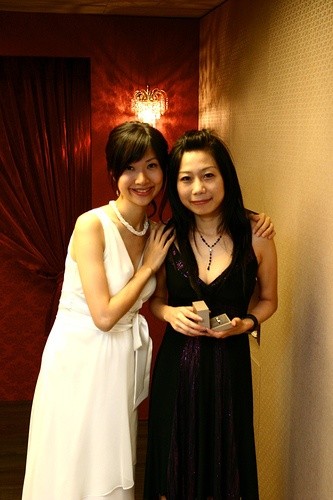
[108,200,149,236]
[196,226,224,271]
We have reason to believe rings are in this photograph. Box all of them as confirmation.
[215,316,221,326]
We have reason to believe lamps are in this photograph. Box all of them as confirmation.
[133,88,168,130]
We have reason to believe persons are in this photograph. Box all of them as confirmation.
[22,121,276,500]
[142,128,279,500]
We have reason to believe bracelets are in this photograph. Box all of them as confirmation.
[245,313,259,332]
